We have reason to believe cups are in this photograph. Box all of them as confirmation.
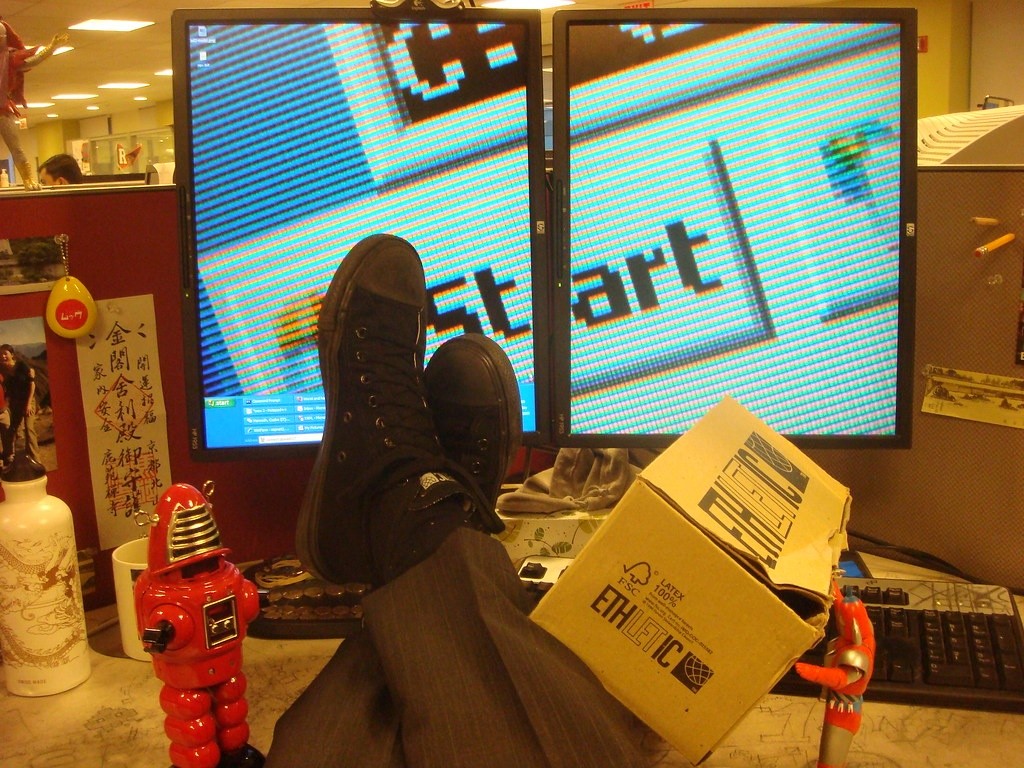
[112,537,153,662]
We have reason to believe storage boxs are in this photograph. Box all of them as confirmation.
[495,507,613,565]
[526,392,852,765]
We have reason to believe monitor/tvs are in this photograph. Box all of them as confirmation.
[171,7,918,464]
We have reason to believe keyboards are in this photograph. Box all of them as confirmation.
[517,554,1024,716]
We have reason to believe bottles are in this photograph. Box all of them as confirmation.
[0,451,91,697]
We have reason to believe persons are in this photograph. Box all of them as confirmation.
[265,233,647,768]
[38,153,83,186]
[0,344,42,466]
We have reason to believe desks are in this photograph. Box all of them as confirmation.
[0,542,1024,768]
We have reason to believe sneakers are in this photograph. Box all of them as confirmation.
[294,234,428,584]
[421,333,523,536]
